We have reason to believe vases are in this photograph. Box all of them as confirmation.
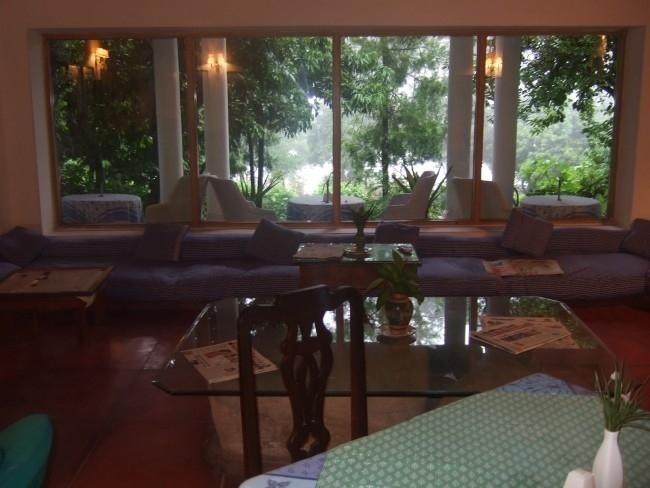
[591,428,624,488]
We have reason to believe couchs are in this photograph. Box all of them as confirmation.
[0,207,650,321]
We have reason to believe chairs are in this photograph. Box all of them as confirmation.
[379,171,436,221]
[145,174,209,221]
[209,177,276,221]
[236,283,368,477]
[452,177,509,218]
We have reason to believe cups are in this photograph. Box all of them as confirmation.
[562,468,598,488]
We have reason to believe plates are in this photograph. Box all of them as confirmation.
[379,324,418,339]
[343,246,371,255]
[295,241,345,261]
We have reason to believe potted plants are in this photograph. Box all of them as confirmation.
[341,201,376,258]
[363,250,424,337]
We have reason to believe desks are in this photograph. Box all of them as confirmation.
[236,372,649,487]
[520,195,601,219]
[286,197,364,223]
[61,193,142,223]
[0,266,112,348]
[290,242,421,290]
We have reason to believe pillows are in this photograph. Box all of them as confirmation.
[619,218,649,255]
[133,222,188,266]
[499,207,553,257]
[0,226,47,263]
[242,216,305,266]
